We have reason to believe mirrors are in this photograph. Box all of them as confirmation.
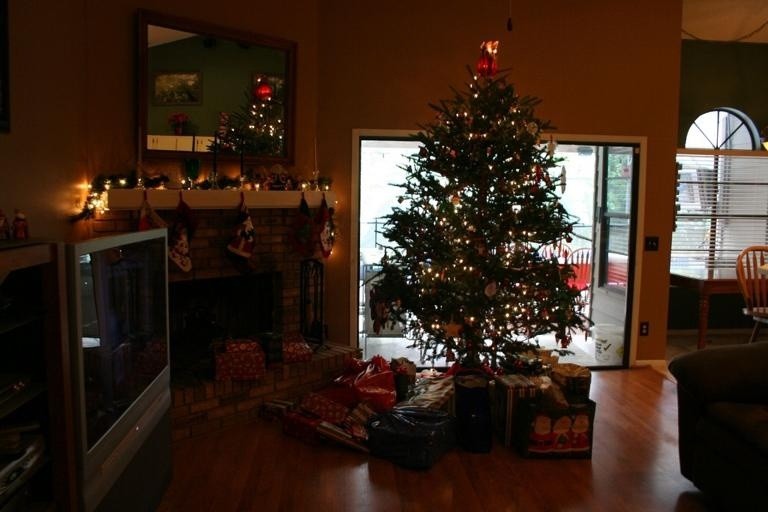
[132,8,298,166]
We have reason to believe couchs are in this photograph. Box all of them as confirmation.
[669,341,768,489]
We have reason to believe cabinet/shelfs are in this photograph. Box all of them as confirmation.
[94,189,338,210]
[0,240,77,512]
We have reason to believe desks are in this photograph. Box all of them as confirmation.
[670,268,768,350]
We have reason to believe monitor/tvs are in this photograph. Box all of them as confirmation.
[48,227,171,512]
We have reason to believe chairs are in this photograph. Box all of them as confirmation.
[736,246,768,344]
[541,242,573,272]
[562,249,591,337]
